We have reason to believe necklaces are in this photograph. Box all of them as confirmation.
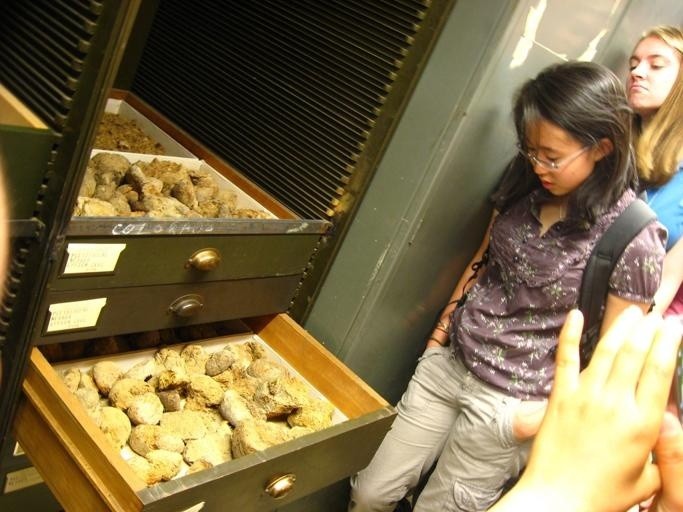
[558,199,571,225]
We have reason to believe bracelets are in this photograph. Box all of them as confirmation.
[422,332,451,347]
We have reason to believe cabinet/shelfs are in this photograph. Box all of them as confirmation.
[2,0,456,511]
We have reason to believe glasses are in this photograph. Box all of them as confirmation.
[515,142,589,173]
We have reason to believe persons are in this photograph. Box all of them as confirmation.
[347,56,666,511]
[624,25,683,411]
[476,300,683,512]
